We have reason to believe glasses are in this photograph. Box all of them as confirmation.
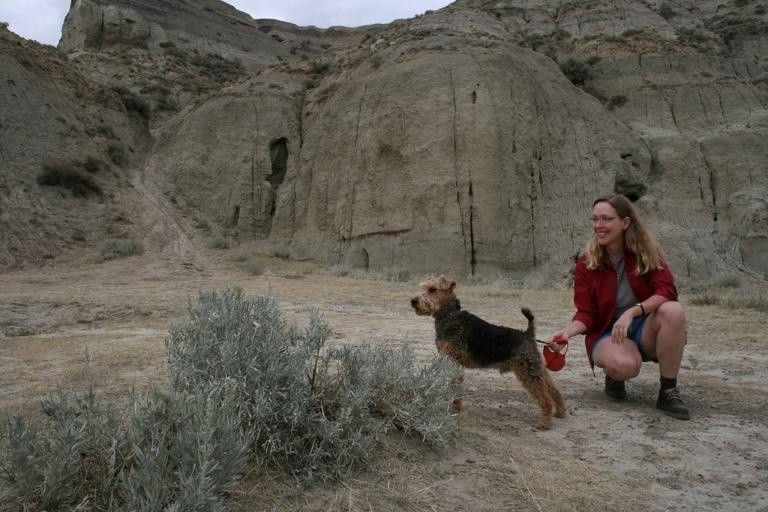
[588,214,616,224]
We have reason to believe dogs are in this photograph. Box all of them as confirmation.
[410,271,567,430]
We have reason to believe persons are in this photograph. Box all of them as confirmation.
[546,194,690,420]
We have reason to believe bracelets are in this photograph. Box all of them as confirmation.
[636,303,644,317]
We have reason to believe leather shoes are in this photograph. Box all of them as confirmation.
[604,375,626,402]
[656,387,690,419]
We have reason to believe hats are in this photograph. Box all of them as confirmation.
[542,339,569,373]
